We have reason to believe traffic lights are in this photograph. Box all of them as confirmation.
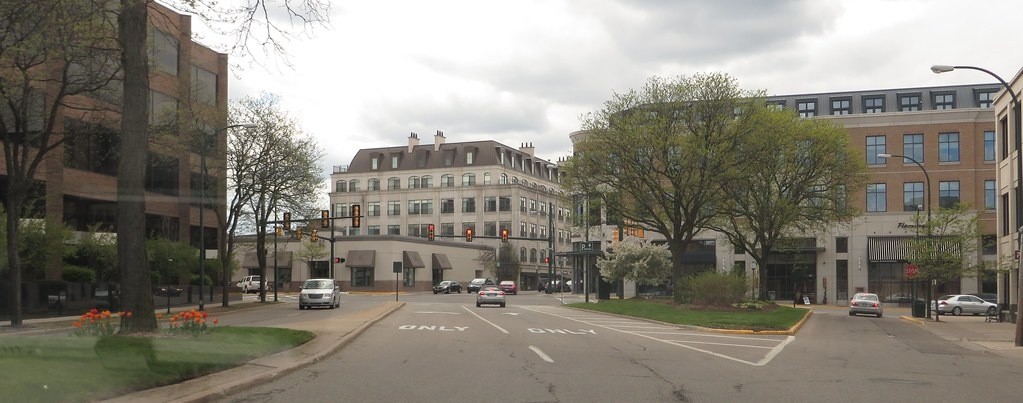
[311,229,318,242]
[335,258,340,263]
[502,230,508,243]
[545,258,549,263]
[297,226,302,240]
[465,229,472,242]
[428,224,434,241]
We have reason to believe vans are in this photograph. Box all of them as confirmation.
[537,278,548,292]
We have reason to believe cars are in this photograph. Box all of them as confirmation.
[299,278,340,310]
[467,278,496,293]
[432,281,462,294]
[496,280,518,295]
[157,284,182,297]
[476,285,505,308]
[545,280,571,294]
[967,292,997,304]
[849,293,883,318]
[566,280,577,292]
[885,292,924,303]
[930,294,997,317]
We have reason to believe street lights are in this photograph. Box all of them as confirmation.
[876,153,932,318]
[751,260,755,300]
[915,204,923,300]
[198,122,259,312]
[929,63,1023,232]
[530,210,557,293]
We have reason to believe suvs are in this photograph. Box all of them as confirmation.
[236,275,268,295]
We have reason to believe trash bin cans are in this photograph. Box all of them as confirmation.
[283,283,291,293]
[912,299,925,318]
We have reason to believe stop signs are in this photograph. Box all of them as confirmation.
[904,263,919,280]
